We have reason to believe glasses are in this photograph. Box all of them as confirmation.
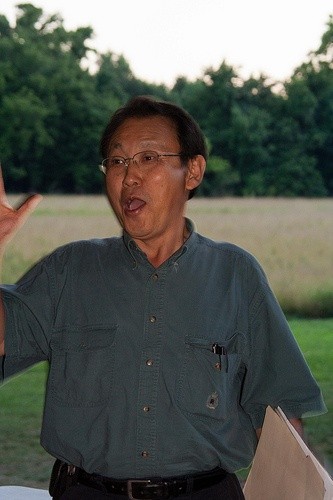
[99,150,181,177]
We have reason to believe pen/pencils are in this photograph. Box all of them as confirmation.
[220,346,227,372]
[213,344,222,372]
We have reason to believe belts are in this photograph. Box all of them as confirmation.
[54,459,228,500]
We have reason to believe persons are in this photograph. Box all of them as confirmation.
[0,95,328,500]
